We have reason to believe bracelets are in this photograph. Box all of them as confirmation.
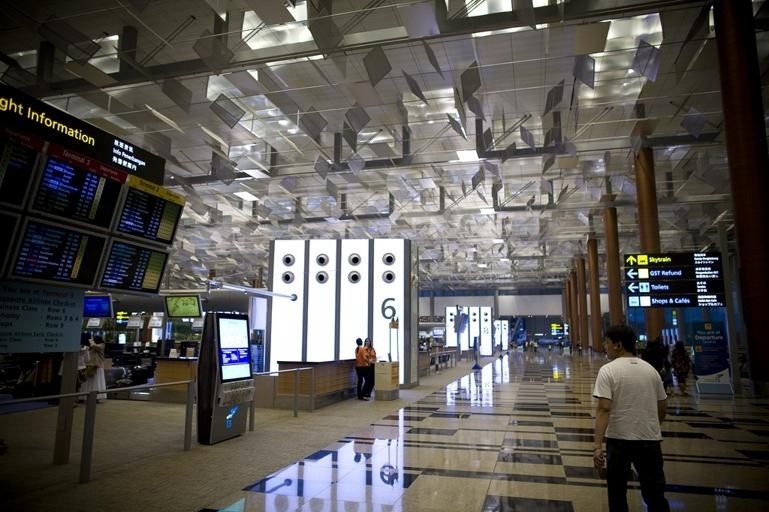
[591,443,603,449]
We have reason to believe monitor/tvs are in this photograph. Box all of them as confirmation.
[157,339,175,355]
[163,293,203,318]
[0,136,185,297]
[215,313,252,382]
[180,341,199,357]
[83,294,114,319]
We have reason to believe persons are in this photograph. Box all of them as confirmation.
[58,333,91,409]
[591,324,670,512]
[355,337,373,402]
[363,337,376,399]
[77,335,108,404]
[641,337,693,398]
[576,341,584,357]
[522,337,574,354]
